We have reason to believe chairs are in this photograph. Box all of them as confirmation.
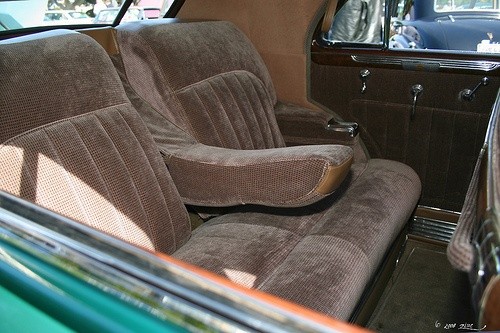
[118,16,421,266]
[1,27,372,324]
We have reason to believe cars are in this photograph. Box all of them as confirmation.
[44,10,95,25]
[93,6,161,26]
[388,0,499,53]
